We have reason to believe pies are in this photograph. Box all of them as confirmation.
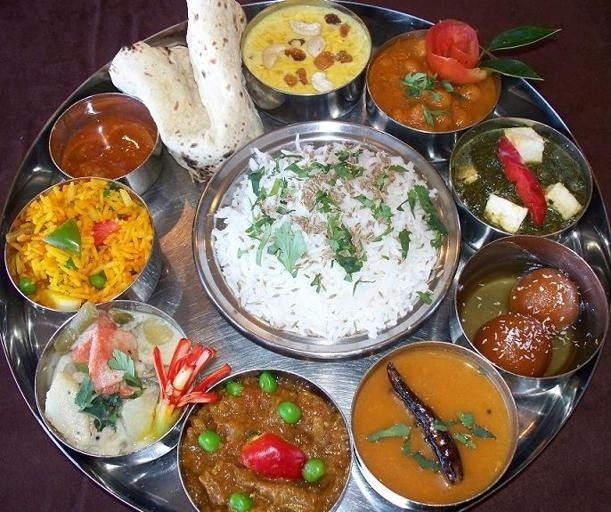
[108,0,265,183]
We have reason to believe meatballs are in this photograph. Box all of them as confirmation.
[315,52,332,70]
[309,37,325,55]
[379,41,480,128]
[476,314,550,377]
[510,264,580,329]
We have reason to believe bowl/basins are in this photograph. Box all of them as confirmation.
[176,368,353,512]
[451,118,592,250]
[34,300,202,466]
[451,235,609,395]
[48,94,164,194]
[241,0,371,123]
[366,29,502,162]
[350,341,519,510]
[5,178,162,324]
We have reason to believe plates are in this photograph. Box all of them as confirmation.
[0,1,611,512]
[193,121,461,361]
